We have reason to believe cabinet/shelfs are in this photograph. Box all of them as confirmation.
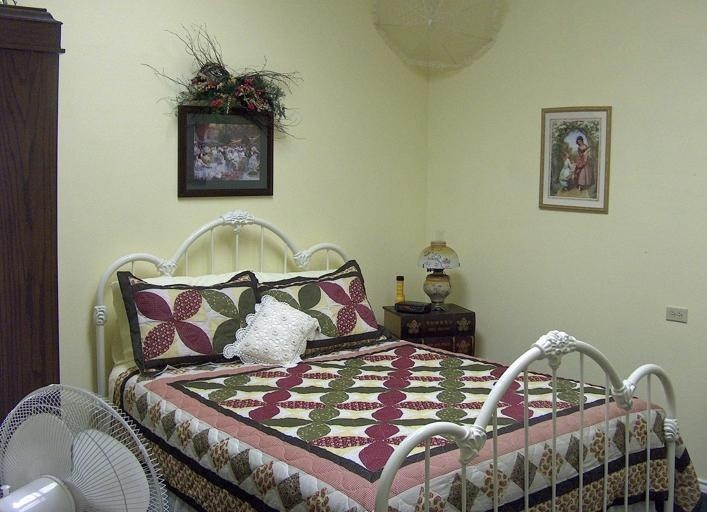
[0,4,61,451]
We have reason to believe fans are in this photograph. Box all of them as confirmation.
[0,384,170,511]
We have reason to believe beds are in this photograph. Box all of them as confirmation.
[93,211,678,511]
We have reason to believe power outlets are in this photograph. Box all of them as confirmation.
[666,306,688,323]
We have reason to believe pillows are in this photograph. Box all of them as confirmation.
[112,260,399,377]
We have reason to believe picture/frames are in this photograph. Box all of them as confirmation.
[178,105,273,197]
[539,106,612,214]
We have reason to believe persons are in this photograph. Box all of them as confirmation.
[194,138,260,181]
[559,153,572,192]
[574,136,592,191]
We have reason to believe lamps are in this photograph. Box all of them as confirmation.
[417,241,460,311]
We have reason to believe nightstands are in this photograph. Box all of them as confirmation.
[382,303,475,356]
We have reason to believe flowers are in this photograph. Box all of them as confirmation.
[173,62,286,116]
